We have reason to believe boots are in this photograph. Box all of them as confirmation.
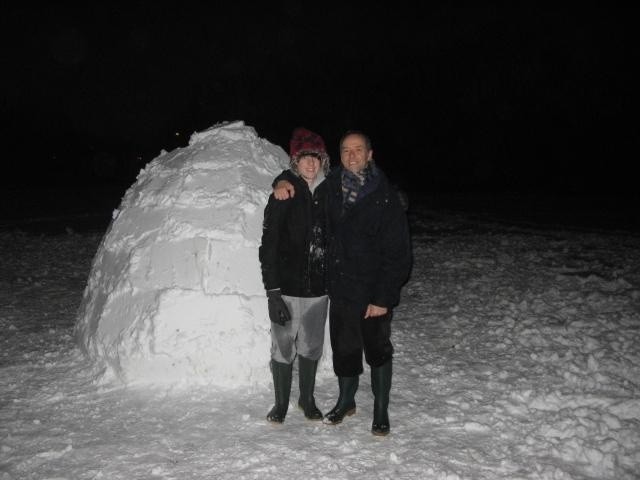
[322,375,360,426]
[369,359,393,436]
[297,353,324,421]
[266,358,295,426]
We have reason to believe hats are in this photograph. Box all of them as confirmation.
[289,128,331,178]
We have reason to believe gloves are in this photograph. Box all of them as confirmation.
[266,288,292,326]
